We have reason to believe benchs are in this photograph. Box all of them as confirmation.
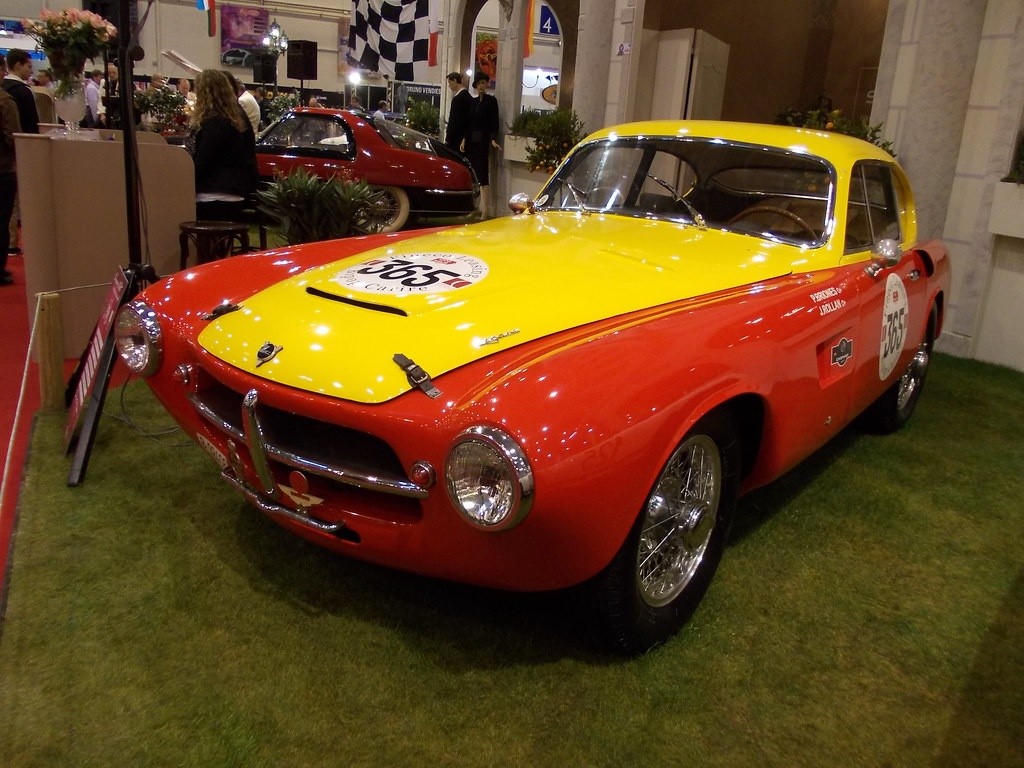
[688,187,891,248]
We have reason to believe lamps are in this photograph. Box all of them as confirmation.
[259,17,289,97]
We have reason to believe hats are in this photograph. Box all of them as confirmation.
[472,71,490,89]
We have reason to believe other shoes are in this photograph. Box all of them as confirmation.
[0,269,13,286]
[9,246,19,256]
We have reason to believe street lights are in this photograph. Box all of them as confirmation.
[263,19,289,97]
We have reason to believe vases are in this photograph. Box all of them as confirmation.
[53,69,89,138]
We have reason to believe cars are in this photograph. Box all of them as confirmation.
[117,117,953,644]
[159,104,480,234]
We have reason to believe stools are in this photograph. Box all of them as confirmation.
[220,205,268,255]
[176,218,252,269]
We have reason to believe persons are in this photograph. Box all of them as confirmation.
[235,77,260,133]
[171,80,197,132]
[256,87,272,131]
[460,72,503,220]
[141,74,170,132]
[184,69,259,261]
[348,96,365,112]
[446,72,473,148]
[86,65,140,130]
[309,98,325,143]
[0,49,53,284]
[373,101,388,120]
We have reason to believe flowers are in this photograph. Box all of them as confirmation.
[19,8,119,97]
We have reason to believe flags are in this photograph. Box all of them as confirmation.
[348,0,438,83]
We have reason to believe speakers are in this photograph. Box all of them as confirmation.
[253,54,275,83]
[286,40,318,79]
